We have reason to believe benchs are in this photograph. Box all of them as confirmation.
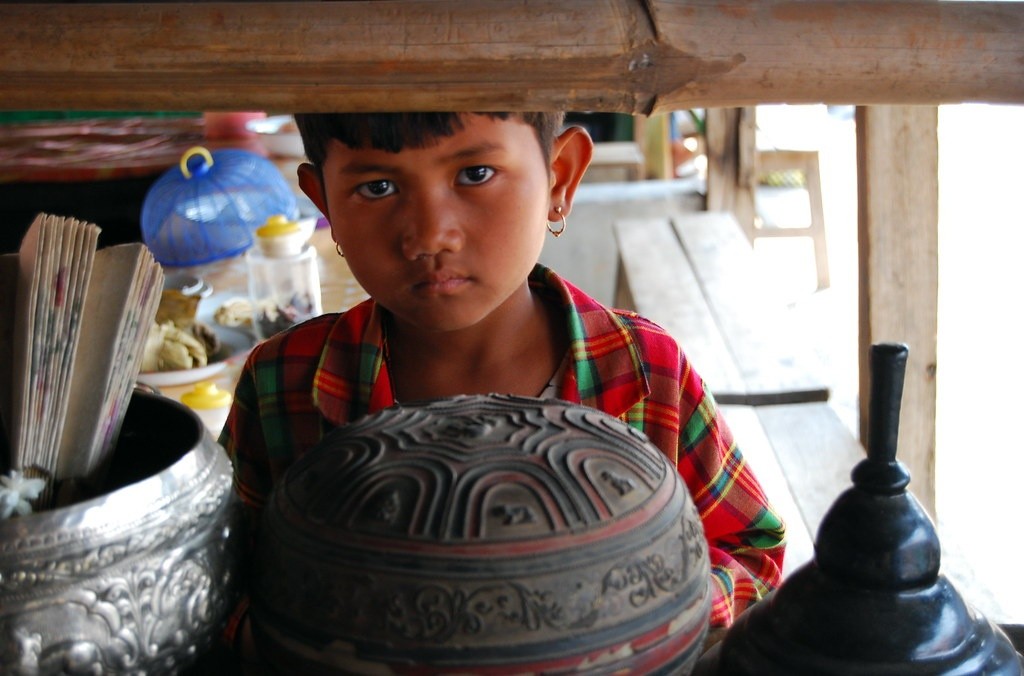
[614,207,870,596]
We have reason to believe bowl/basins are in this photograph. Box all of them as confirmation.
[257,218,318,255]
[136,324,260,442]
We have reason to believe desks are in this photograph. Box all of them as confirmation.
[0,115,312,186]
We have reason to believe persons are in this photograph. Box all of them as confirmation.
[218,109,788,661]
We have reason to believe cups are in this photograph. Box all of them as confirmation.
[245,246,323,339]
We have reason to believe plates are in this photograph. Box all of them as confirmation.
[161,274,214,298]
